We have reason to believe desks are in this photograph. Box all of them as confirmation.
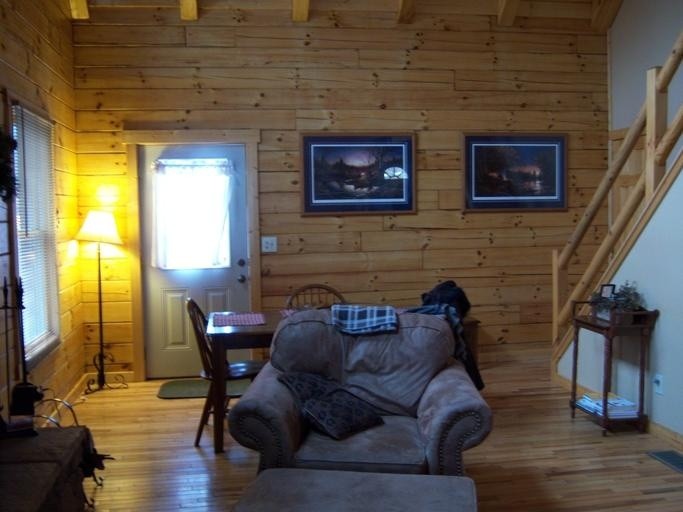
[462,316,480,327]
[206,311,286,453]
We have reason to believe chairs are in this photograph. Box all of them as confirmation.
[227,308,493,476]
[286,284,348,309]
[185,298,267,447]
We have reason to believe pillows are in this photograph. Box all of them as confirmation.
[276,371,384,440]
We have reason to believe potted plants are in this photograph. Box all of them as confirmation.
[584,279,645,323]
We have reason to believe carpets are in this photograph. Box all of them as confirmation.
[156,378,250,398]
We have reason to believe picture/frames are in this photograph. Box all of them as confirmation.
[298,132,420,218]
[460,131,569,213]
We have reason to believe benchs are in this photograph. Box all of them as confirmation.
[0,425,92,512]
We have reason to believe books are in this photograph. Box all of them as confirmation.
[575,391,638,419]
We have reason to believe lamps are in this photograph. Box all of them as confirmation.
[73,209,129,395]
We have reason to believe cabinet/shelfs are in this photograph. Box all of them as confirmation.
[568,298,659,436]
